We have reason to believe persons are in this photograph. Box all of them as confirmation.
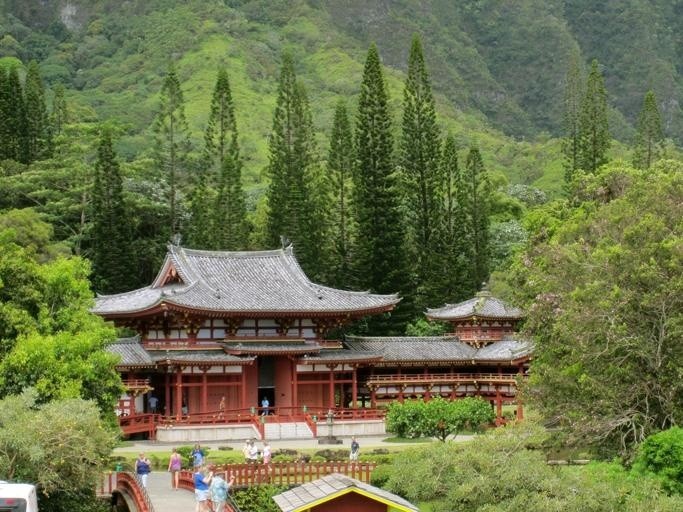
[261,397,269,416]
[167,447,181,491]
[242,440,272,472]
[134,451,152,488]
[147,393,158,414]
[217,396,226,419]
[193,464,235,512]
[350,436,360,470]
[190,442,205,469]
